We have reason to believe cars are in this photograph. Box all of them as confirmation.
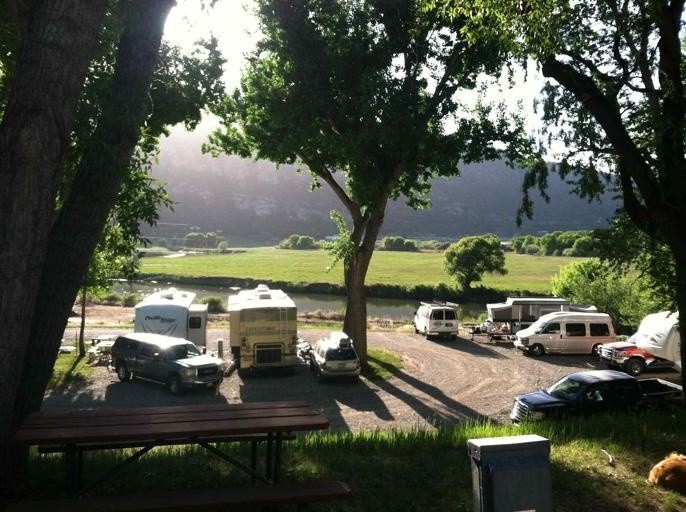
[309,330,362,382]
[596,331,676,376]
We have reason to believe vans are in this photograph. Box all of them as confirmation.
[512,311,616,358]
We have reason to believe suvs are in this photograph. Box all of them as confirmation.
[412,299,461,340]
[110,331,225,396]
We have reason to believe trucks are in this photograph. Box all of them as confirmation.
[227,282,310,373]
[133,286,209,348]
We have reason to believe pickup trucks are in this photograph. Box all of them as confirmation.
[509,368,684,423]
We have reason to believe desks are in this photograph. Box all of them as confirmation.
[10,381,329,511]
[471,333,515,343]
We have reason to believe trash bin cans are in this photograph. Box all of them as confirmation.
[467,435,551,512]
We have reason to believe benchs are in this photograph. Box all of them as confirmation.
[10,431,351,510]
